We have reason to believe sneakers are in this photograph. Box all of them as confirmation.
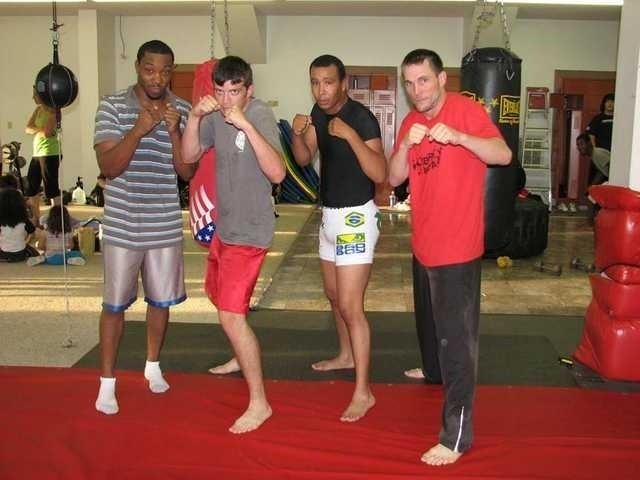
[27,255,45,266]
[67,256,86,266]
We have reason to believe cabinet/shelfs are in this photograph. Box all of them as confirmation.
[345,65,398,208]
[169,63,197,107]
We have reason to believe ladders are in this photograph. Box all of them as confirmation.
[520,87,553,212]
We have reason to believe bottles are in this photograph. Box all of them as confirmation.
[388,191,397,207]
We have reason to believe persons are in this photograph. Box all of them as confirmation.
[583,92,615,196]
[289,52,388,424]
[90,39,199,416]
[0,172,87,267]
[575,133,611,178]
[23,84,64,230]
[179,54,288,436]
[386,47,513,466]
